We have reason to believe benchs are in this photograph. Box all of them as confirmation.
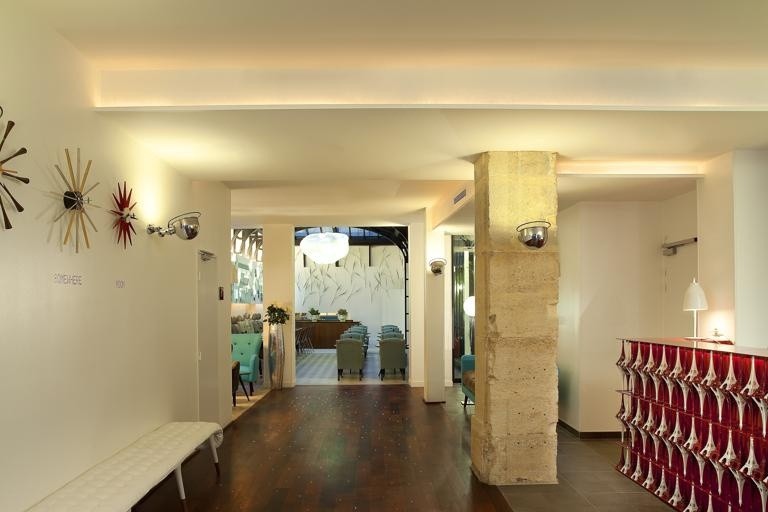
[27,420,224,512]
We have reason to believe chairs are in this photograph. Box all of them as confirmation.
[295,326,315,358]
[231,333,262,396]
[335,326,406,381]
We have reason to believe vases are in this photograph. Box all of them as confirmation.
[267,323,285,391]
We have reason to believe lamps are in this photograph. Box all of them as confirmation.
[515,221,551,249]
[682,279,709,341]
[148,211,201,240]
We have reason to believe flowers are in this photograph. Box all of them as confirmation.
[263,300,293,326]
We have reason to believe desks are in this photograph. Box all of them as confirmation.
[296,320,361,349]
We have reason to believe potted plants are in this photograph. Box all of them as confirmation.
[308,308,348,322]
